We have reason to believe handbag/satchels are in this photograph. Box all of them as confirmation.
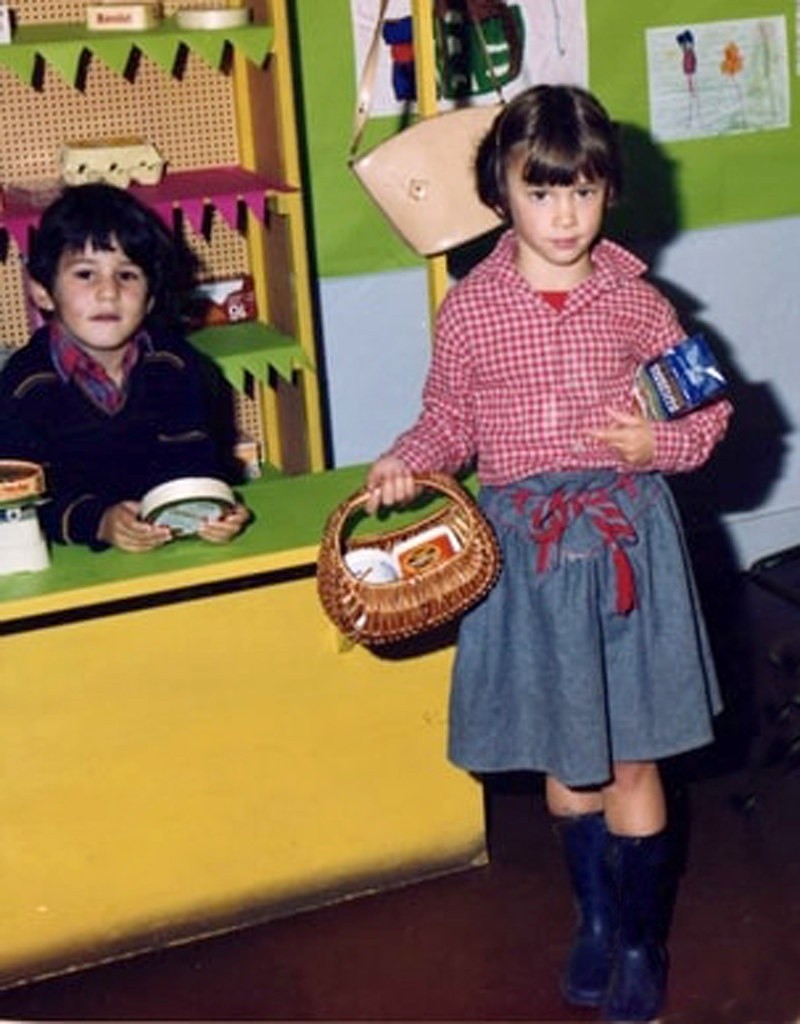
[343,0,509,260]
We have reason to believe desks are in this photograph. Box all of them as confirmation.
[3,445,482,991]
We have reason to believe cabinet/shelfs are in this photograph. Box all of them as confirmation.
[0,0,323,486]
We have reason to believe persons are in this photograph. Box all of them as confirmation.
[365,86,733,1024]
[1,181,251,553]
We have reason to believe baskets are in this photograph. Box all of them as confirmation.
[317,475,503,649]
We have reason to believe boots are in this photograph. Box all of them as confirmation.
[605,821,678,1020]
[544,800,615,1008]
